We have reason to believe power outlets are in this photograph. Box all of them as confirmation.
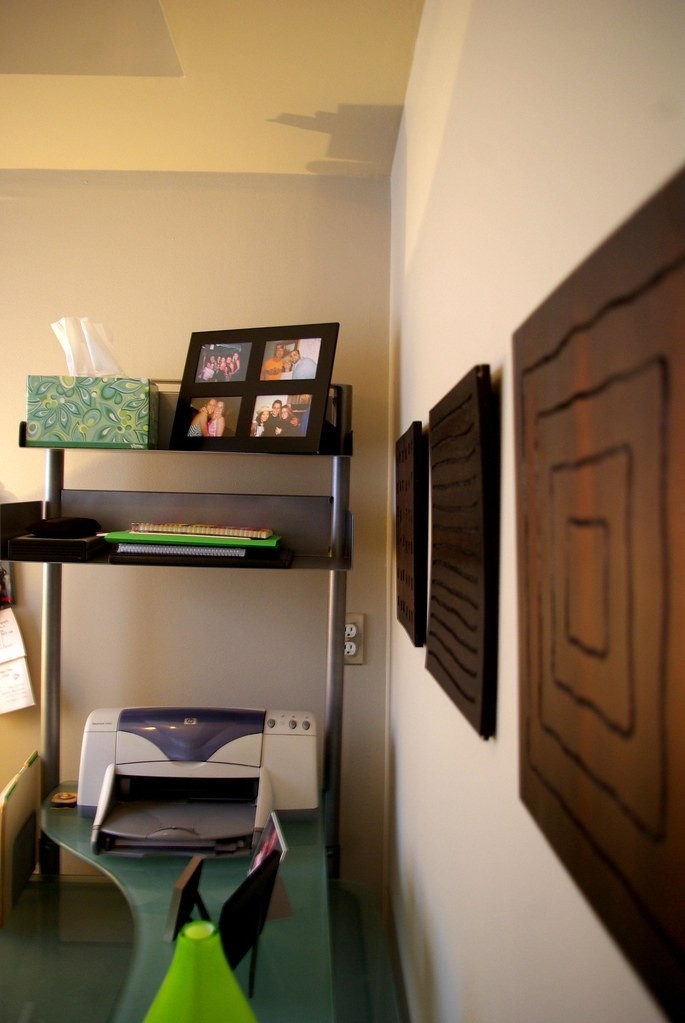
[343,612,365,666]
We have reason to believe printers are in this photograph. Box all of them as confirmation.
[78,709,322,859]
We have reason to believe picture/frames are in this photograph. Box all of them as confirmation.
[167,321,341,453]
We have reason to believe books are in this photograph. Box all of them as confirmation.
[98,522,283,556]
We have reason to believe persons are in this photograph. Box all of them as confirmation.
[187,399,225,437]
[198,352,241,382]
[260,343,317,381]
[250,400,300,436]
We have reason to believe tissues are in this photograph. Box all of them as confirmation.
[24,315,159,450]
